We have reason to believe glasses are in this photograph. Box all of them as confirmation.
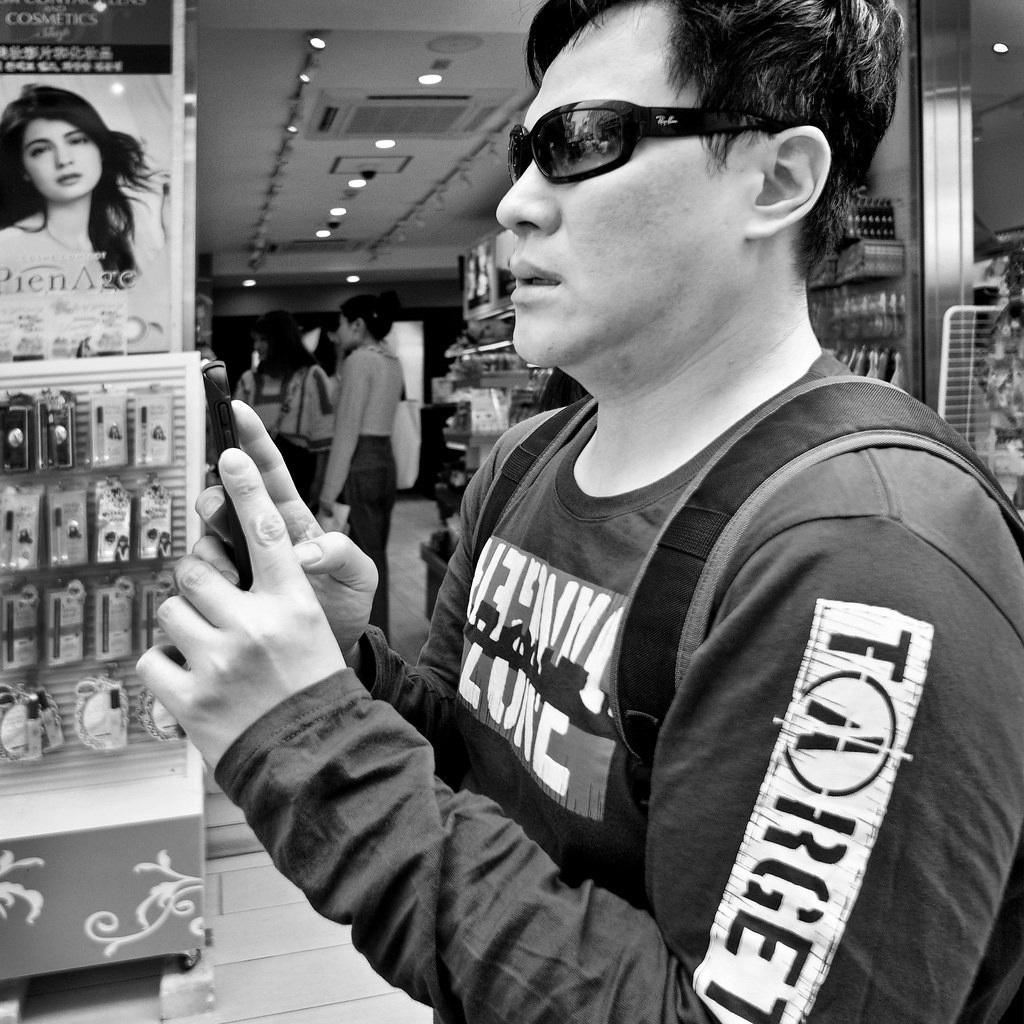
[507,100,789,185]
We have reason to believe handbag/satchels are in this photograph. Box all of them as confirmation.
[389,386,422,490]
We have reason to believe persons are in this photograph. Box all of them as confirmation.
[138,0,1024,1024]
[232,288,402,647]
[1,87,171,291]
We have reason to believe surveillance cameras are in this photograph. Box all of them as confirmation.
[327,218,341,229]
[358,164,378,181]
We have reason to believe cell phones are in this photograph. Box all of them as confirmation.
[200,359,254,592]
